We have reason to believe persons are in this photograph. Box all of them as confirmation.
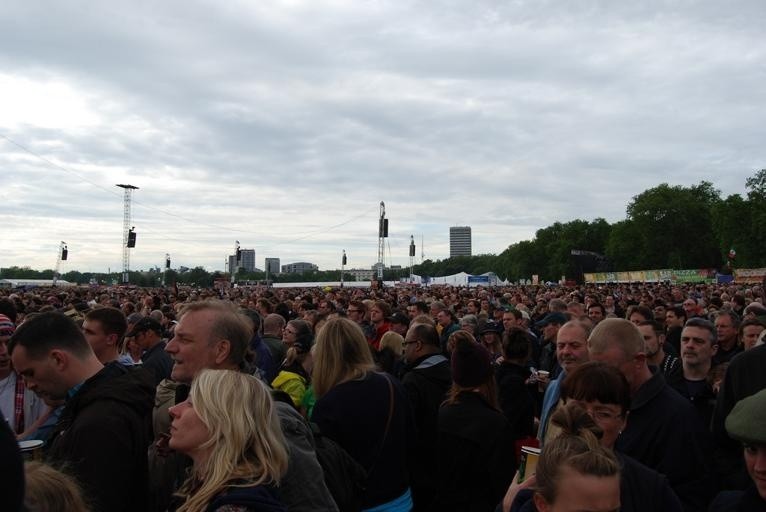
[435,282,530,360]
[2,412,87,512]
[262,314,289,364]
[538,320,593,445]
[530,281,766,327]
[636,321,679,376]
[500,325,540,436]
[164,301,342,512]
[705,386,766,512]
[740,315,765,349]
[528,312,565,416]
[496,363,684,512]
[716,312,744,360]
[588,318,718,511]
[128,318,176,386]
[0,314,50,434]
[666,318,716,414]
[533,403,620,511]
[171,370,291,512]
[712,341,765,490]
[79,309,126,367]
[1,281,196,323]
[195,279,351,317]
[438,340,516,510]
[273,329,315,409]
[306,317,416,512]
[9,310,155,512]
[281,318,312,342]
[237,307,272,375]
[346,279,435,367]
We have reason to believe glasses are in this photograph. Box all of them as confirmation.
[403,340,424,346]
[371,310,384,314]
[283,328,297,335]
[494,306,503,309]
[581,409,625,420]
[346,308,360,313]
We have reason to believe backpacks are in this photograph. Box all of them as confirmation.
[270,389,368,512]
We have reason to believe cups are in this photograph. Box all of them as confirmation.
[538,371,549,393]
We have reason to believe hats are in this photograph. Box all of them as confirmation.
[569,291,581,296]
[742,303,764,315]
[480,323,496,334]
[521,311,530,319]
[386,312,410,324]
[536,311,565,326]
[447,331,493,388]
[127,312,143,323]
[126,317,164,337]
[652,299,669,307]
[726,388,766,444]
[503,293,512,298]
[323,287,331,291]
